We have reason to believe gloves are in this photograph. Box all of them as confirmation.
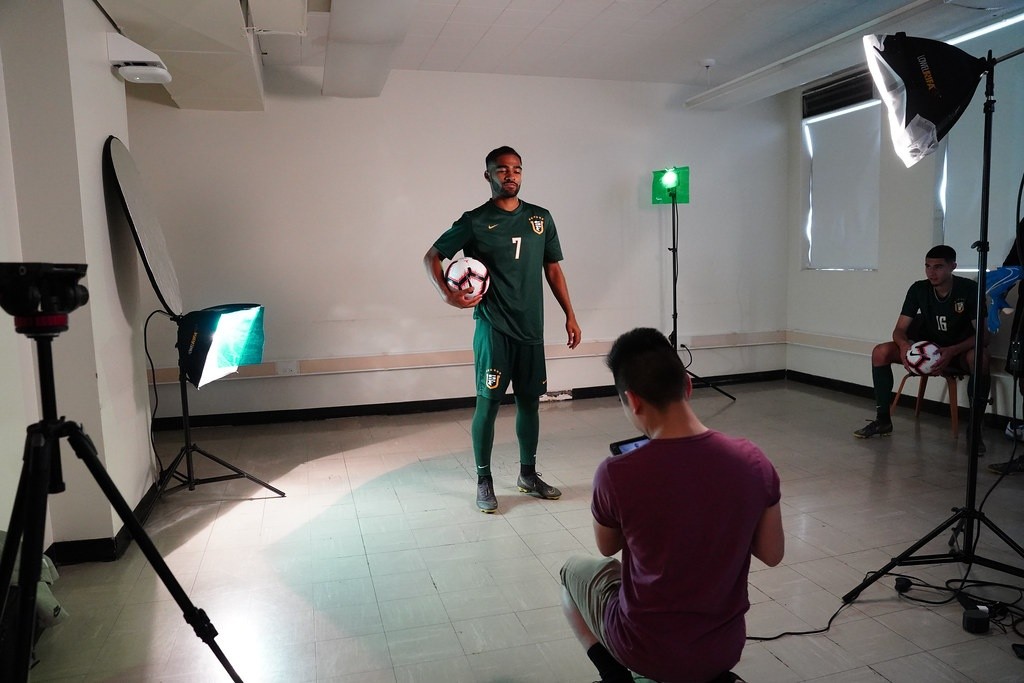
[987,295,1014,336]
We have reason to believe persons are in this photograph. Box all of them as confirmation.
[424,145,581,513]
[559,328,786,683]
[988,217,1024,473]
[853,245,990,456]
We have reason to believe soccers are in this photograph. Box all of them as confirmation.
[905,340,943,377]
[445,257,490,301]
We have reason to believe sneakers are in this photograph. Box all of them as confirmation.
[475,477,499,515]
[1005,422,1024,442]
[851,421,895,439]
[987,454,1023,476]
[515,473,562,500]
[967,425,986,457]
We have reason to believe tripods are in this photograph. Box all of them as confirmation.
[668,191,735,405]
[1,265,242,681]
[147,317,285,500]
[842,56,1021,604]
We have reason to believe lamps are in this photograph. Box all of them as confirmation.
[141,303,285,522]
[841,31,1024,603]
[651,167,689,351]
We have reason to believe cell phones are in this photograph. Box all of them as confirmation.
[611,433,652,458]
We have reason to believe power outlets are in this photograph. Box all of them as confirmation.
[677,336,691,350]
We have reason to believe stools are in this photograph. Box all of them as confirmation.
[890,373,962,439]
